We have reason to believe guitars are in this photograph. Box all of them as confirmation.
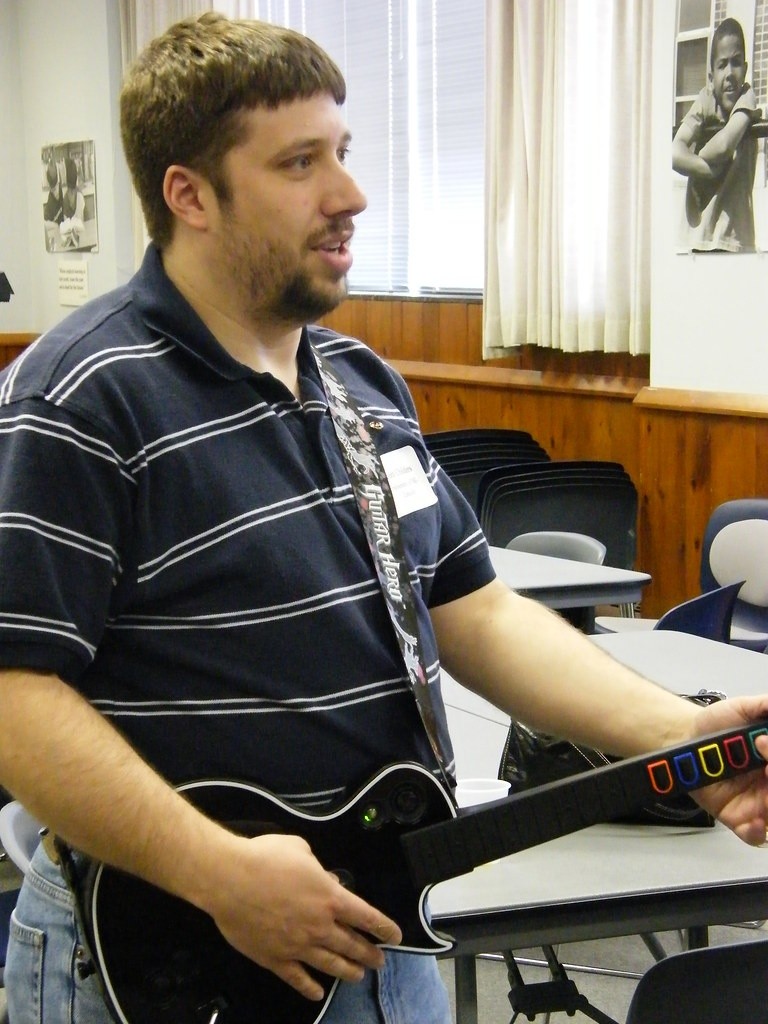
[80,715,767,1024]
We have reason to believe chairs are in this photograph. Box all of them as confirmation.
[505,531,607,635]
[624,939,768,1024]
[653,577,747,645]
[593,498,768,653]
[422,428,639,618]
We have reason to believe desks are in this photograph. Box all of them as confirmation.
[428,630,768,1024]
[487,546,652,619]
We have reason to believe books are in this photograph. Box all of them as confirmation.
[65,228,79,247]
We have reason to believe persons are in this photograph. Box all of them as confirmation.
[43,159,89,224]
[670,19,762,255]
[0,14,768,1024]
